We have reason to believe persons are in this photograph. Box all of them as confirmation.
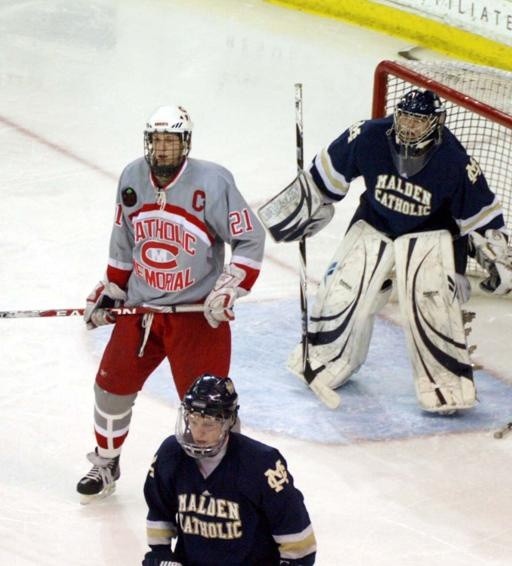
[76,105,265,495]
[142,373,317,566]
[258,90,512,415]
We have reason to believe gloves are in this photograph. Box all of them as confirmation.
[83,280,130,332]
[142,552,187,566]
[202,263,264,329]
[467,228,512,297]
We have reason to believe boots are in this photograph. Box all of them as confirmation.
[77,447,121,495]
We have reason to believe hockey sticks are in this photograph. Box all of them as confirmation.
[294,83,341,409]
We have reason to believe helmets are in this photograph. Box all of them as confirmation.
[386,89,447,129]
[143,103,194,157]
[181,372,239,429]
